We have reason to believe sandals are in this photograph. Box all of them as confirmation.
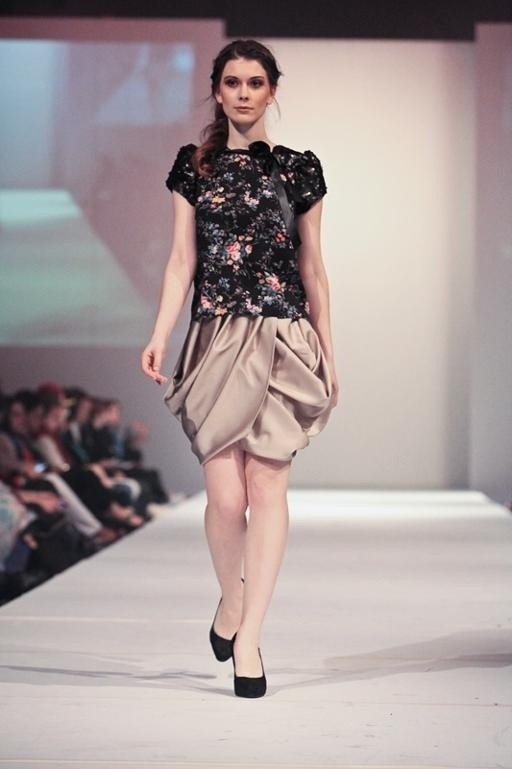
[210,577,245,663]
[232,645,267,699]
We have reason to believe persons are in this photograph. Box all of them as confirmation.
[139,40,339,697]
